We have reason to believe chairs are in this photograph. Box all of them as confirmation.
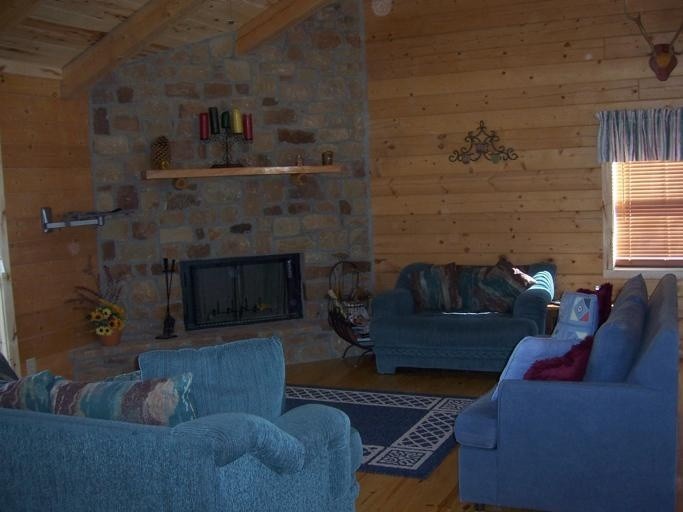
[369,262,557,373]
[327,261,376,368]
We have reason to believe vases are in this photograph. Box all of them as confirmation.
[100,329,121,346]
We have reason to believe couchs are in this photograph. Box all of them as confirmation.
[0,407,362,512]
[454,272,679,512]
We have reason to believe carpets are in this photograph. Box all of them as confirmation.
[284,384,478,479]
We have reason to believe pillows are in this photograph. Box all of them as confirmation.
[474,256,536,313]
[137,335,285,421]
[405,261,462,311]
[50,370,196,428]
[0,353,55,411]
[491,272,648,401]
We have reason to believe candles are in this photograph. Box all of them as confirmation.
[242,113,253,140]
[221,111,229,128]
[199,112,210,139]
[208,107,220,134]
[230,106,243,134]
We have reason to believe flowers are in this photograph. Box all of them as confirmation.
[64,255,128,335]
